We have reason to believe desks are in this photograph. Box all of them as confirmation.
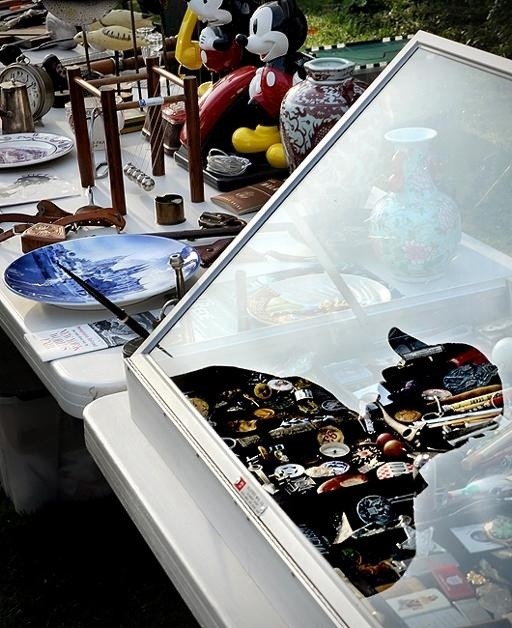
[0,72,261,418]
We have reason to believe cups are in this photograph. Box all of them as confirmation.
[154,194,183,224]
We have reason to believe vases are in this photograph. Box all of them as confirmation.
[368,128,463,285]
[280,56,367,176]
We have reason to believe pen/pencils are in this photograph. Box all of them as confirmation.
[58,261,172,359]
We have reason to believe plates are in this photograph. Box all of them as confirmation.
[246,273,392,325]
[2,234,200,310]
[0,133,74,168]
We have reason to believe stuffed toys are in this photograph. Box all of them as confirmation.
[186,0,244,98]
[230,0,308,169]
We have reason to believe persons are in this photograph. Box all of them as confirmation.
[169,327,504,597]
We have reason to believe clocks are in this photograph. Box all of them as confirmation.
[0,62,55,127]
